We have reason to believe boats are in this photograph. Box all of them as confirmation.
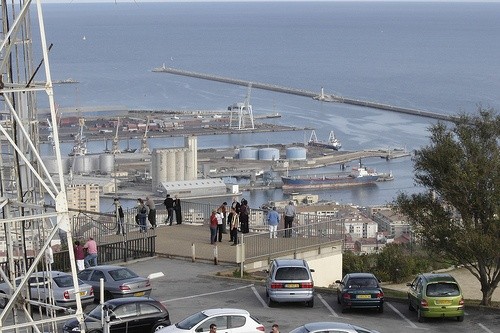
[68,117,137,157]
[280,163,380,185]
[308,129,342,150]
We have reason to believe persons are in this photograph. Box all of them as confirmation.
[231,208,239,246]
[231,198,249,233]
[174,195,181,224]
[283,201,295,238]
[145,198,157,229]
[74,241,85,271]
[164,194,173,225]
[267,207,280,239]
[114,198,124,236]
[215,207,224,242]
[220,201,227,233]
[137,199,145,214]
[84,237,98,267]
[210,211,218,244]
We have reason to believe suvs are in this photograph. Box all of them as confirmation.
[263,258,315,307]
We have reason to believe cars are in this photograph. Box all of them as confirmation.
[0,271,94,316]
[66,265,152,304]
[290,322,382,333]
[62,297,171,333]
[407,273,465,322]
[335,272,384,313]
[154,308,265,333]
